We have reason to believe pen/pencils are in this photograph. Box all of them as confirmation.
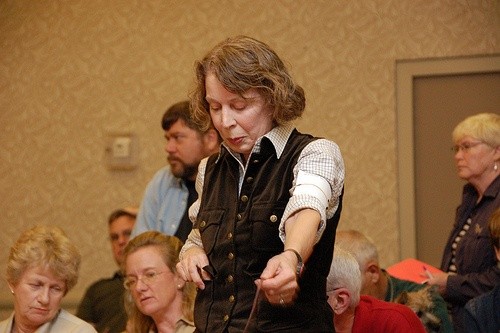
[423,265,432,279]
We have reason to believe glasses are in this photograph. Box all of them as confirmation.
[124,268,173,289]
[449,140,483,155]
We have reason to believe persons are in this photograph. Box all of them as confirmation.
[335,229,453,333]
[327,245,428,333]
[0,224,98,333]
[75,208,138,333]
[120,231,197,333]
[176,34,346,333]
[420,112,500,333]
[128,100,224,244]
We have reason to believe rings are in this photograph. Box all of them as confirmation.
[280,299,286,306]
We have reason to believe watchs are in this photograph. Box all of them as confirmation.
[284,249,307,279]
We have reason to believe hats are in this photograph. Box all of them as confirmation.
[107,207,139,222]
